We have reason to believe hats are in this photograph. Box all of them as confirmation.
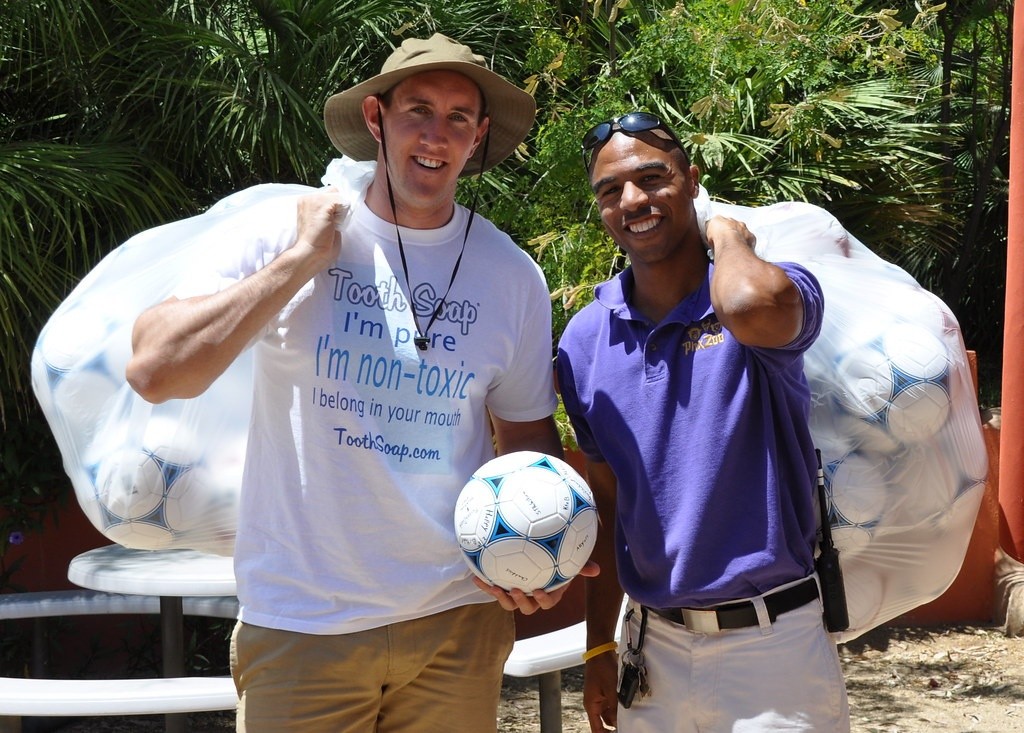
[324,32,536,177]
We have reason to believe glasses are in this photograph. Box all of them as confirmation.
[581,112,691,173]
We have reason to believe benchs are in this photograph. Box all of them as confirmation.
[0,584,595,733]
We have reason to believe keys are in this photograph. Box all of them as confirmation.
[617,665,638,710]
[629,661,652,702]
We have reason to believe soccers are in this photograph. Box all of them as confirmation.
[452,451,600,598]
[750,199,992,644]
[30,184,251,557]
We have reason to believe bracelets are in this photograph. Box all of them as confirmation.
[583,641,618,661]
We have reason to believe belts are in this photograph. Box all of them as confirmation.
[643,578,819,632]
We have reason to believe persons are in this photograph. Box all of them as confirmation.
[125,32,600,733]
[556,110,852,733]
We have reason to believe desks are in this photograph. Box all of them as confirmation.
[67,546,238,732]
